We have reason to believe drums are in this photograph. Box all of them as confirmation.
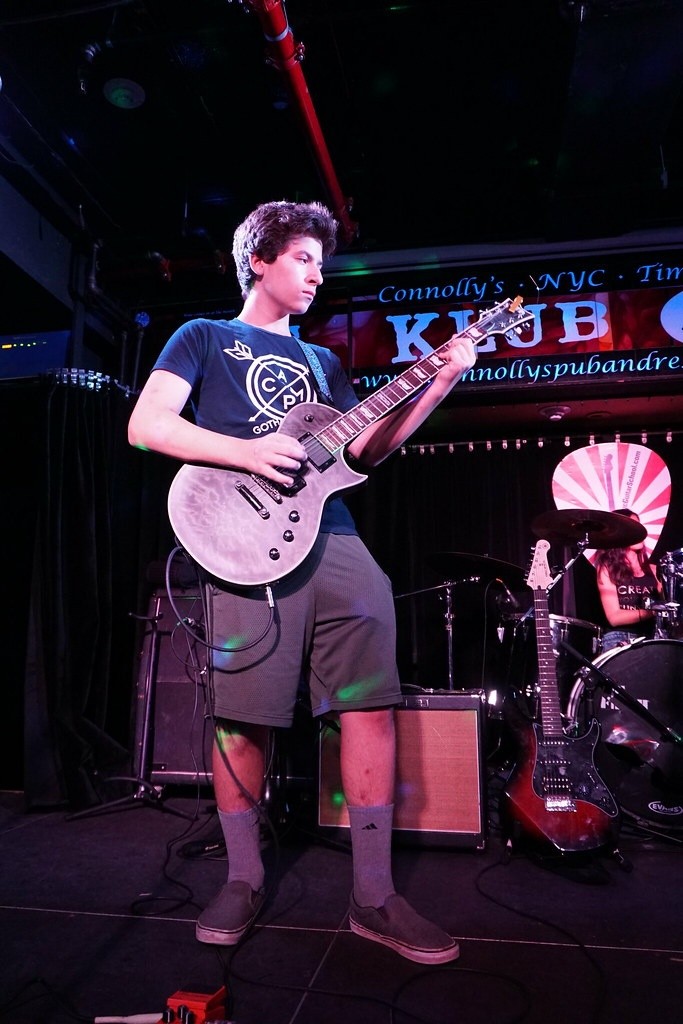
[497,612,603,699]
[566,638,683,830]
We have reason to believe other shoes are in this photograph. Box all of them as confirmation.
[348,889,459,965]
[196,880,267,946]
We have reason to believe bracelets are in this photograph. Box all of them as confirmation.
[638,608,642,622]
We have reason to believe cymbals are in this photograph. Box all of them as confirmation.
[432,552,528,587]
[529,509,648,548]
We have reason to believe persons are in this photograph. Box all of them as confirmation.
[487,583,506,616]
[127,201,479,965]
[594,508,666,655]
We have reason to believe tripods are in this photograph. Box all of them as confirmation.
[62,597,197,822]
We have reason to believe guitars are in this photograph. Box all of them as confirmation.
[505,538,619,853]
[168,294,535,586]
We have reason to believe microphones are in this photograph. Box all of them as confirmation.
[185,616,205,631]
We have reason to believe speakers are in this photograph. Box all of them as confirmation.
[134,588,275,788]
[314,694,485,849]
